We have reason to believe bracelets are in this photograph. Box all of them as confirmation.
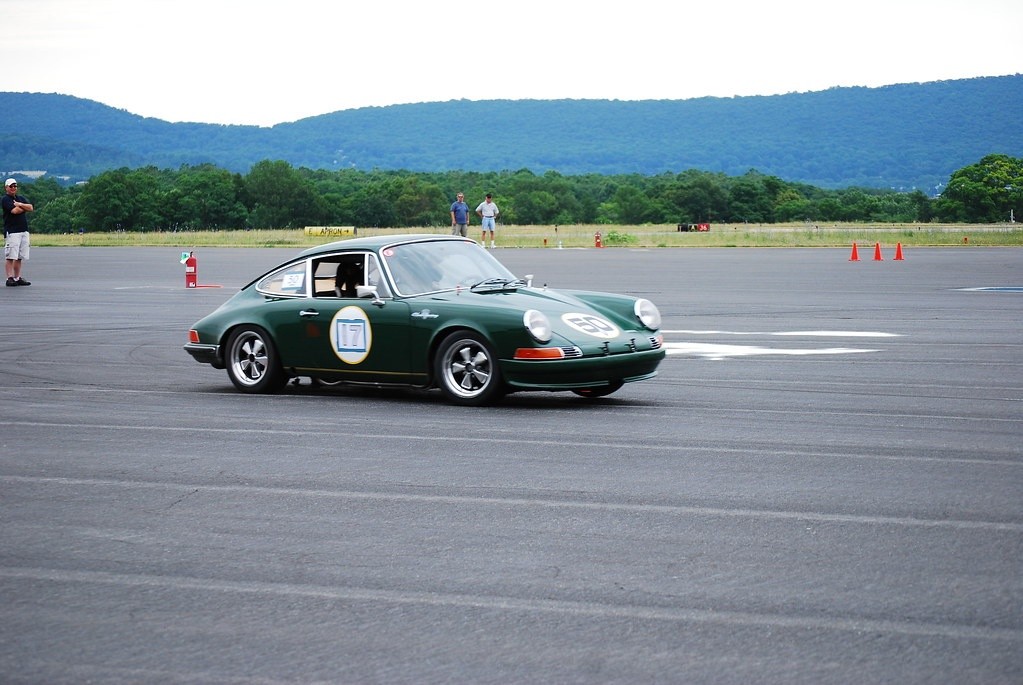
[19,203,22,207]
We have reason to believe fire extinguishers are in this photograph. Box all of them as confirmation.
[186,251,197,288]
[595,230,601,247]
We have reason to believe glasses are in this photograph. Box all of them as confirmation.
[9,185,17,188]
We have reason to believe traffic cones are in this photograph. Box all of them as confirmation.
[871,242,885,262]
[848,243,862,262]
[892,242,904,261]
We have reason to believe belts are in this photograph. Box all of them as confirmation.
[483,216,494,218]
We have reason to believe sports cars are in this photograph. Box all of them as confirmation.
[181,232,669,408]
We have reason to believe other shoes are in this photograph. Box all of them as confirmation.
[491,245,496,249]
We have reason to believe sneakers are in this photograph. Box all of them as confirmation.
[16,276,31,286]
[6,277,17,286]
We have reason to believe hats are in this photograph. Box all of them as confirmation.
[486,194,492,198]
[5,179,17,186]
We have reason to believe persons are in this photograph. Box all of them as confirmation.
[0,178,33,286]
[335,260,365,297]
[451,193,469,237]
[476,194,498,248]
